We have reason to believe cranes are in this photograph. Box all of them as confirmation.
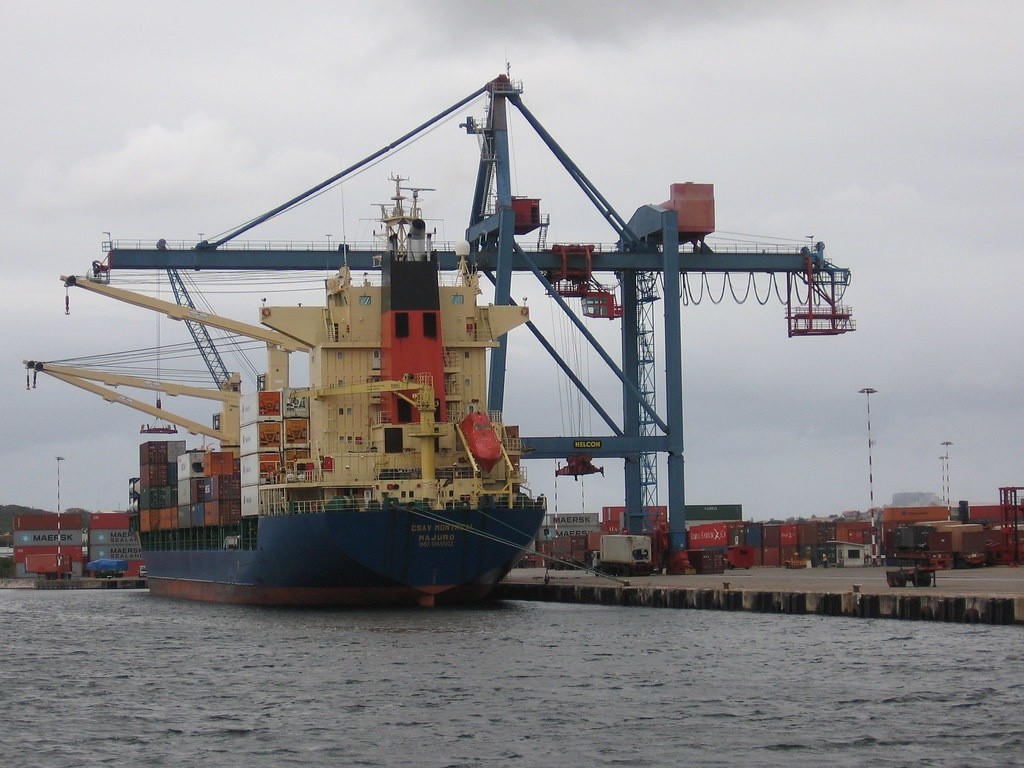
[102,61,860,576]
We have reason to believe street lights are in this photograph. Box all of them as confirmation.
[856,388,882,568]
[940,442,954,521]
[939,456,950,506]
[54,456,65,580]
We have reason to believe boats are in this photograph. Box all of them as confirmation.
[21,173,554,613]
[460,411,503,474]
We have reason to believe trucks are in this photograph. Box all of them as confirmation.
[591,534,651,578]
[883,525,953,588]
